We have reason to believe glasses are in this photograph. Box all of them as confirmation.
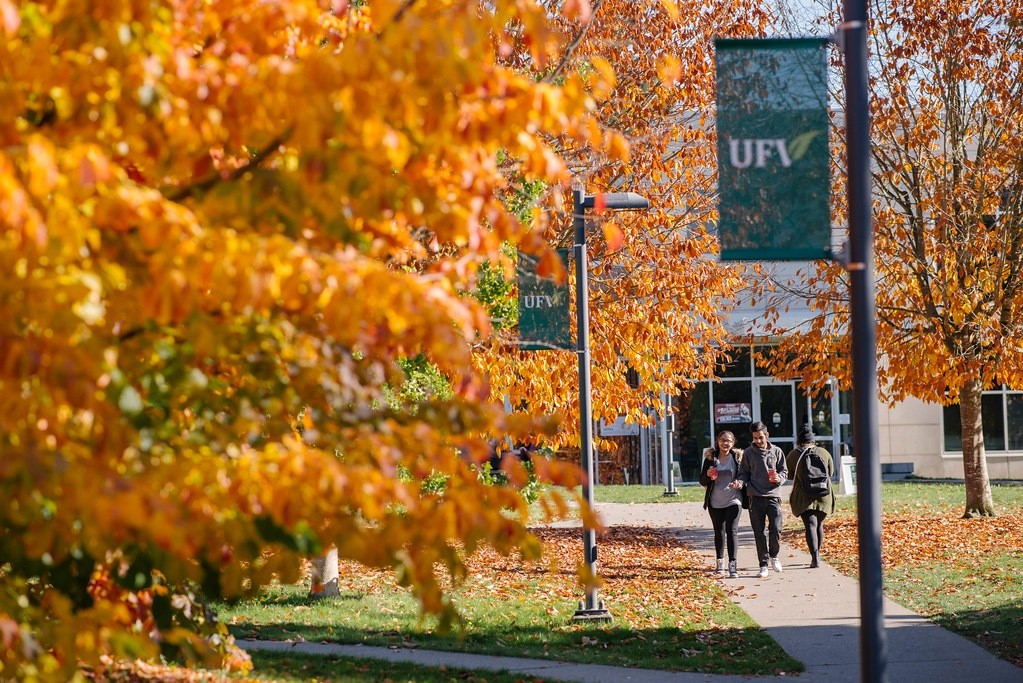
[719,438,733,443]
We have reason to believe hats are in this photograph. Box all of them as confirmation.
[798,424,814,441]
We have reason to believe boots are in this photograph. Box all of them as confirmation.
[810,549,820,568]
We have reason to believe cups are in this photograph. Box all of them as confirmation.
[768,468,777,482]
[709,467,718,480]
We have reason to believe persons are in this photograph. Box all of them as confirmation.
[699,430,745,580]
[785,424,837,569]
[731,421,789,579]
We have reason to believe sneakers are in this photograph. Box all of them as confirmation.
[716,558,725,576]
[757,567,768,577]
[729,560,739,578]
[771,558,782,572]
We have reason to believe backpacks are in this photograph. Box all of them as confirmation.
[795,447,830,499]
